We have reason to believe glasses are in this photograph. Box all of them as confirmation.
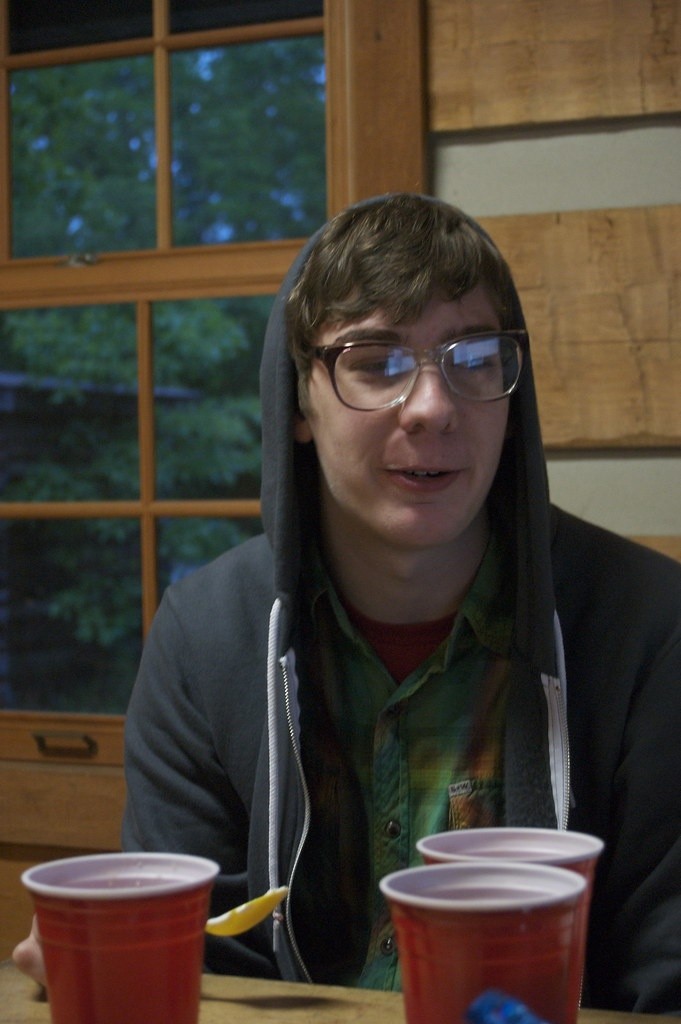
[305,327,522,413]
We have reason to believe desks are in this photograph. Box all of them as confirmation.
[1,970,681,1024]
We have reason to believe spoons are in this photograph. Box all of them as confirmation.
[203,886,291,936]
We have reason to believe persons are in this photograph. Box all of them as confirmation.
[11,194,681,1024]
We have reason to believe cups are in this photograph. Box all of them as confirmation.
[21,850,220,1024]
[379,863,590,1024]
[416,826,604,887]
